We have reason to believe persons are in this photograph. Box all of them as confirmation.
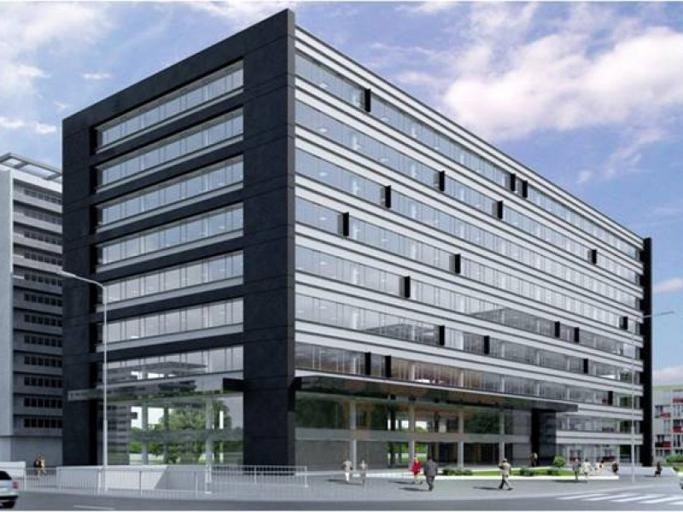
[498,458,513,490]
[572,458,581,481]
[581,458,593,482]
[358,457,368,486]
[34,454,48,478]
[424,455,438,491]
[409,455,423,484]
[341,456,355,484]
